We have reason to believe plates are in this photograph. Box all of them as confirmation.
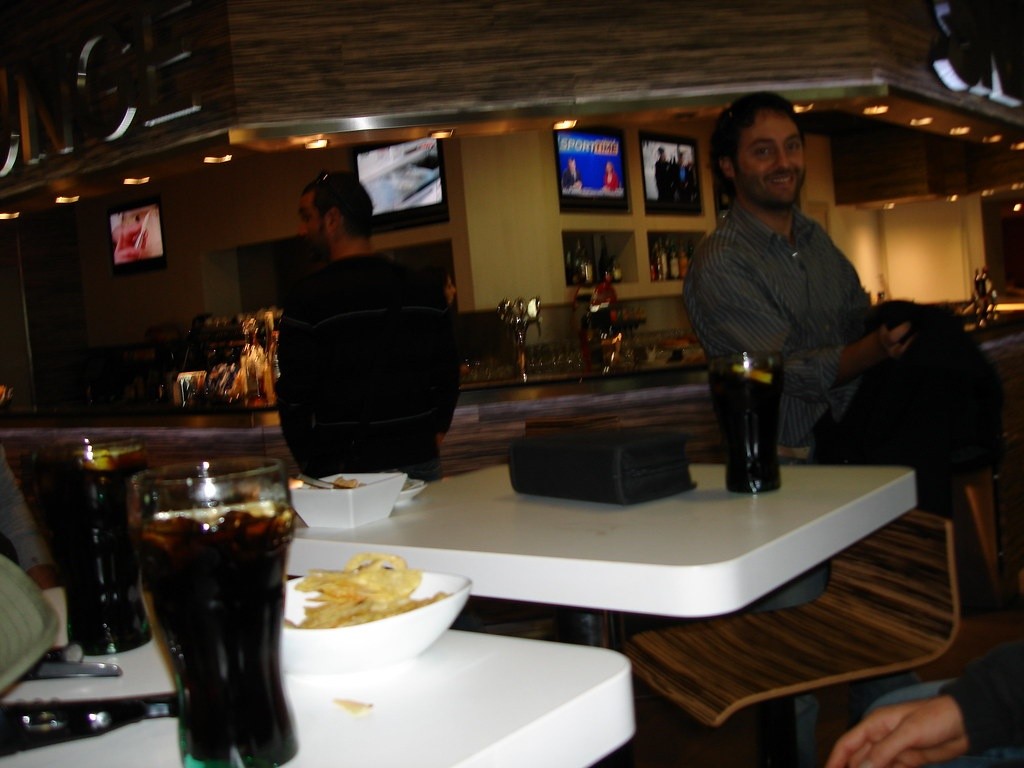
[396,474,426,511]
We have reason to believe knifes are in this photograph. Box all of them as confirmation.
[25,660,122,680]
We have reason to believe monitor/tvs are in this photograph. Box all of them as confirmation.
[108,196,168,277]
[553,126,631,213]
[353,136,450,233]
[638,133,703,217]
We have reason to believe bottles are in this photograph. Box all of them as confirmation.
[650,238,696,282]
[565,233,623,287]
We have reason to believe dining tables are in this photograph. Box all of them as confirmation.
[286,464,918,768]
[0,588,636,768]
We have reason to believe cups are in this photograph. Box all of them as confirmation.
[710,353,784,492]
[130,457,298,768]
[37,438,152,655]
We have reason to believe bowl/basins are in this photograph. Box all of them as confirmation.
[292,472,406,528]
[276,570,472,670]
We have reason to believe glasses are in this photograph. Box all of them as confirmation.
[316,170,354,215]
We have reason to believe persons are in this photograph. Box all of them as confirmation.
[274,171,487,636]
[0,444,61,653]
[564,148,700,214]
[118,215,148,258]
[682,90,1023,768]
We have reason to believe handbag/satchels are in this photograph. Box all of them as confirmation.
[509,430,696,504]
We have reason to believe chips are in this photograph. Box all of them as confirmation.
[280,552,454,628]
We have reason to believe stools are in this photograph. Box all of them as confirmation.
[627,512,963,768]
